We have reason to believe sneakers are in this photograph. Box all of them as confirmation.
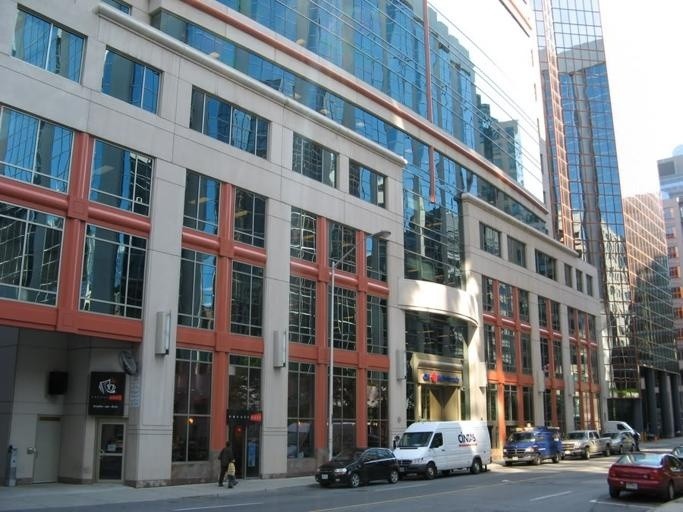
[219,482,239,488]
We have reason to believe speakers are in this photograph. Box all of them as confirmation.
[48,371,68,395]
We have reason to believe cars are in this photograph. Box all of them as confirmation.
[316,446,400,488]
[607,452,683,500]
[600,433,636,455]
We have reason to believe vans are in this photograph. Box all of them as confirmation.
[392,420,492,480]
[608,421,640,439]
[503,425,564,465]
[561,430,603,459]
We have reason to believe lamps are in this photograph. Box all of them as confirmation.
[272,329,288,372]
[153,308,172,356]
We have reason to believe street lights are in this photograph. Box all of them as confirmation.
[329,231,391,462]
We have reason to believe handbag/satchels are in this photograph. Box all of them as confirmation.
[227,462,236,477]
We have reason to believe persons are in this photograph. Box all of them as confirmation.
[633,432,640,451]
[226,457,237,489]
[393,435,400,449]
[217,440,240,487]
